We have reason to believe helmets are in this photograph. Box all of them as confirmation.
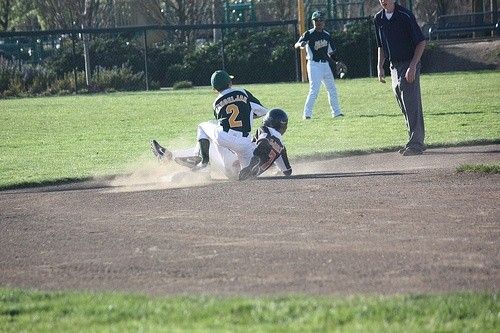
[263,108,288,136]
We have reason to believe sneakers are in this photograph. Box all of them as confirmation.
[151,140,173,167]
[239,155,262,180]
[190,161,210,173]
[174,156,201,168]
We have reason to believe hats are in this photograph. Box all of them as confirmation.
[311,10,326,20]
[211,70,234,88]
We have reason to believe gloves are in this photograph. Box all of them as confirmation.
[336,61,347,75]
[299,31,310,47]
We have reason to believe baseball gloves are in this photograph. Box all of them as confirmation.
[336,62,347,78]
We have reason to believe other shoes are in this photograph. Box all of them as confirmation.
[334,114,344,117]
[398,143,426,154]
[303,116,310,120]
[402,147,422,155]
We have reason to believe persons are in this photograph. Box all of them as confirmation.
[151,108,292,182]
[190,70,268,181]
[294,11,346,119]
[374,0,426,155]
